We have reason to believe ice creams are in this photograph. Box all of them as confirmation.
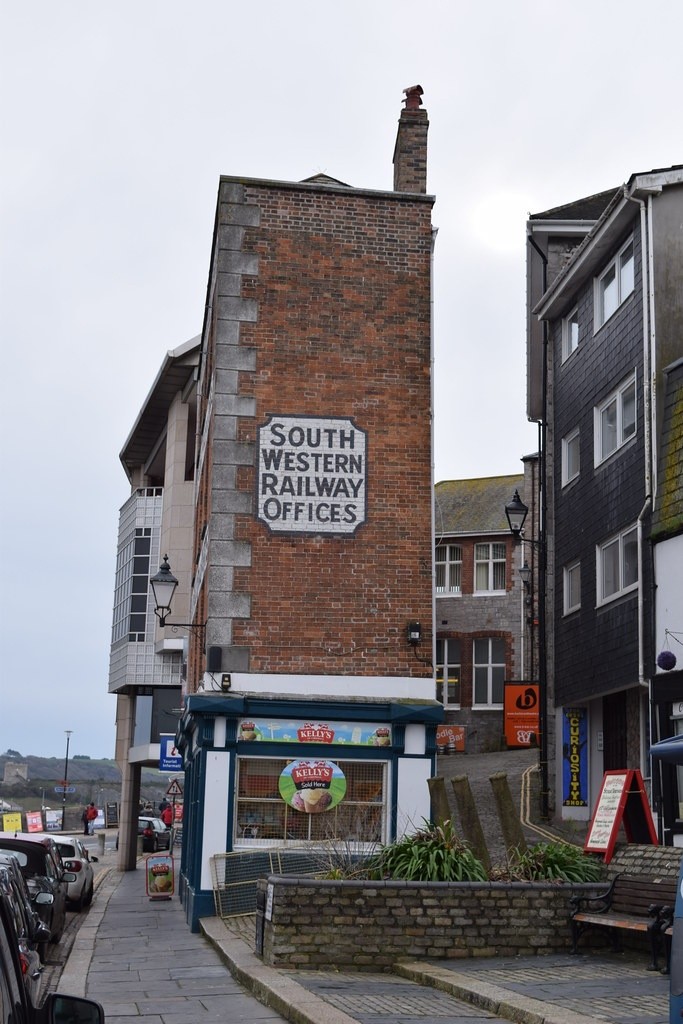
[154,875,167,892]
[300,787,323,813]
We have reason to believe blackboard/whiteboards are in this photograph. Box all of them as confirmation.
[105,801,119,825]
[584,769,634,853]
[173,827,183,844]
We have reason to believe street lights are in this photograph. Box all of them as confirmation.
[39,787,45,809]
[61,730,75,829]
[95,788,103,805]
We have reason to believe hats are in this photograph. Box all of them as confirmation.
[90,802,94,806]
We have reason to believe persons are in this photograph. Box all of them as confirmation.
[159,798,170,812]
[159,805,173,827]
[141,804,153,817]
[81,802,98,835]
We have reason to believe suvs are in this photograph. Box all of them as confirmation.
[0,829,72,947]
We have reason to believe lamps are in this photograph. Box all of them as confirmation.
[518,562,534,604]
[149,555,206,655]
[506,489,549,552]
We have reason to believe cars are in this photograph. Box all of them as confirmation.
[42,833,98,909]
[0,850,105,1024]
[115,817,171,852]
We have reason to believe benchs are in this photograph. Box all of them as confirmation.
[567,870,680,974]
